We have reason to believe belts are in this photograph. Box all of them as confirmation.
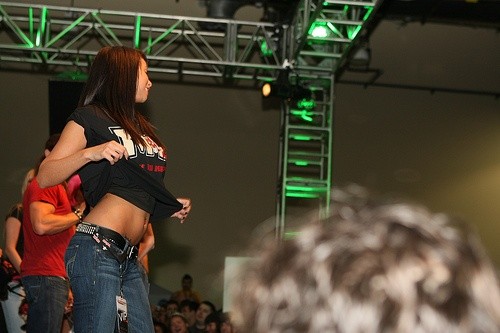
[75,221,140,262]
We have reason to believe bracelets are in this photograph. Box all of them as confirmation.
[74,209,84,219]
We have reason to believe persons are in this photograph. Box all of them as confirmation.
[236,189,500,333]
[0,133,235,333]
[37,46,191,333]
